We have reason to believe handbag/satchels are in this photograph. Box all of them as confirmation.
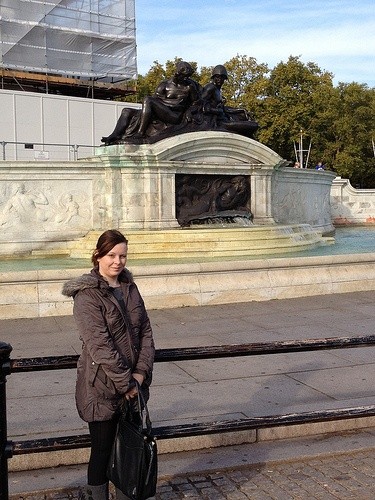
[105,378,158,500]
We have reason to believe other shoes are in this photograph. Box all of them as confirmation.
[86,481,130,500]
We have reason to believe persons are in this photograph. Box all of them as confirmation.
[100,61,258,144]
[315,161,325,169]
[207,177,251,213]
[61,229,156,499]
